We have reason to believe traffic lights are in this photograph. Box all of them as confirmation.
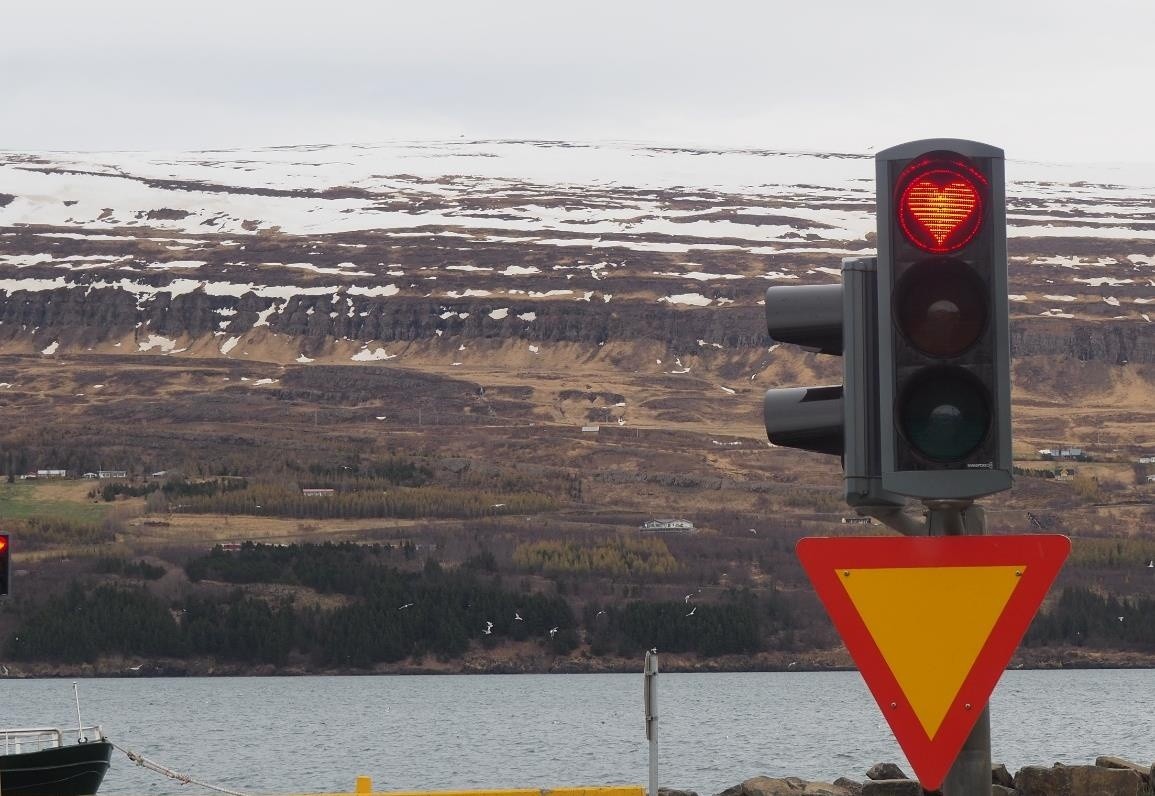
[0,531,11,597]
[763,255,879,517]
[874,137,1011,502]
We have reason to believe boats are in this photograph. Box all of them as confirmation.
[0,682,115,796]
